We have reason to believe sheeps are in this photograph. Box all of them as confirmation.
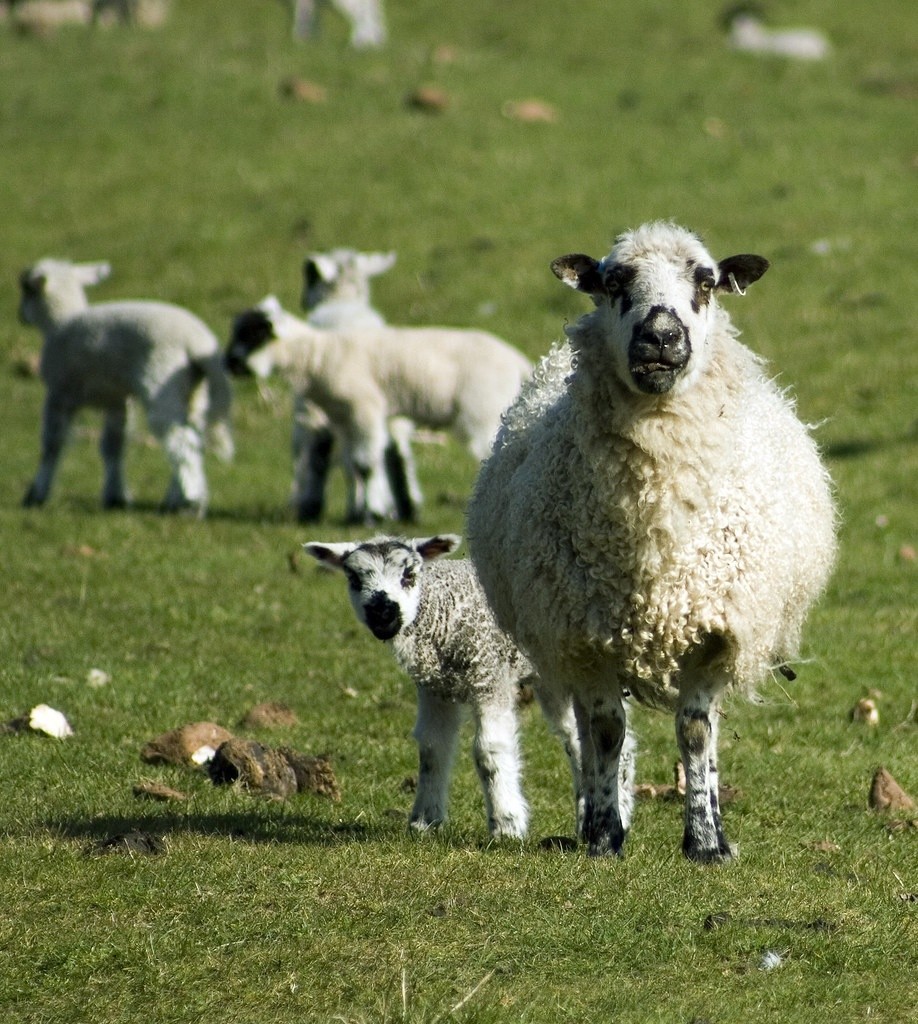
[225,245,536,529]
[467,217,845,863]
[13,256,233,521]
[298,533,584,851]
